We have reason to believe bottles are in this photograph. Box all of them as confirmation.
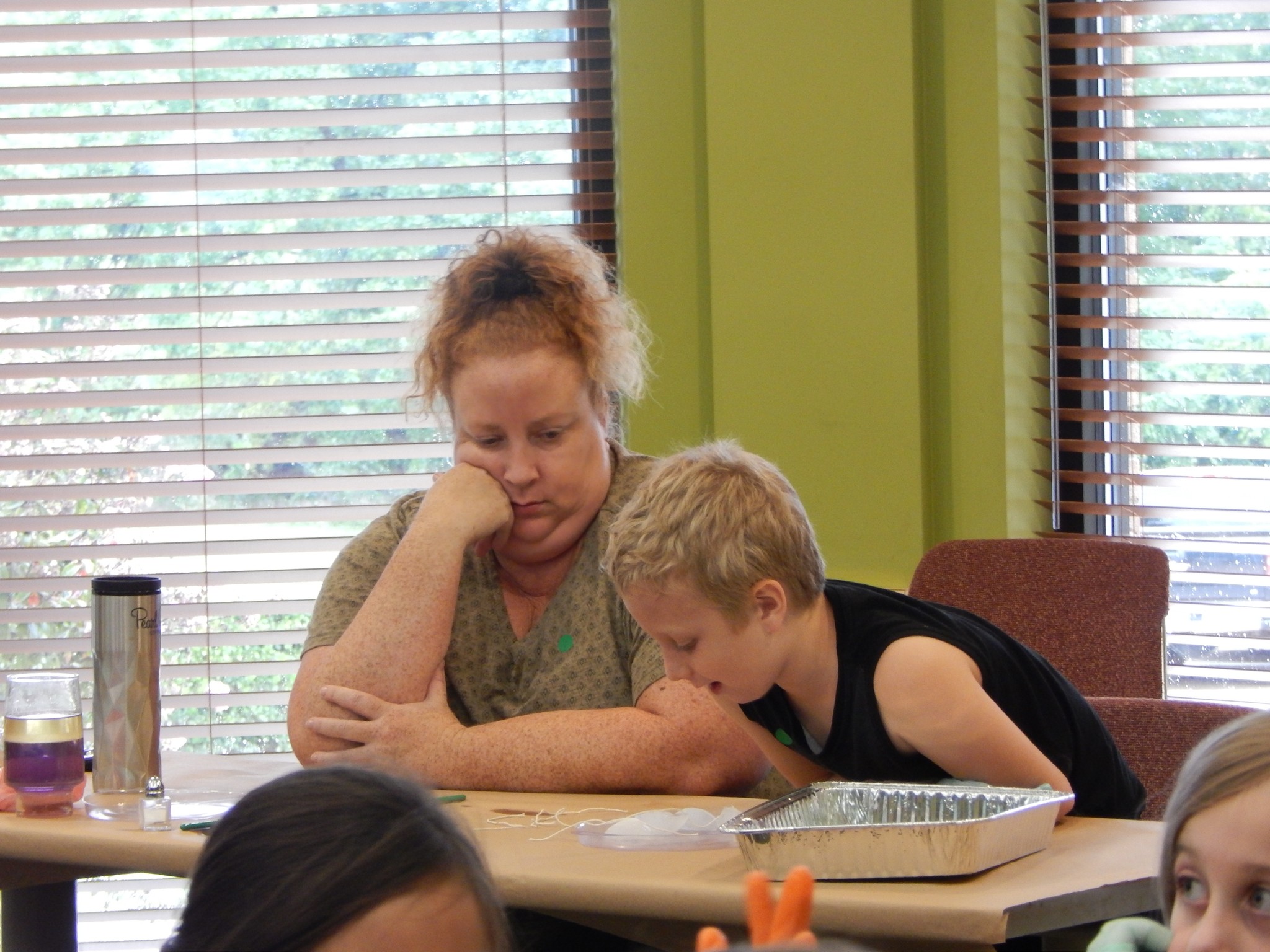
[140,774,173,831]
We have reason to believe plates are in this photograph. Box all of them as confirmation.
[571,826,741,850]
[82,791,245,822]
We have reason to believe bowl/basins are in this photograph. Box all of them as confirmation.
[719,780,1075,882]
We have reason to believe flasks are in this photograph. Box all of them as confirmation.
[92,575,162,794]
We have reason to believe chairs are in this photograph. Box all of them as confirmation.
[908,537,1170,698]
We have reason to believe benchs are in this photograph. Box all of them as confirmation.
[1084,697,1260,821]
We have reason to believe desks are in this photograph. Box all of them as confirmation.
[0,771,1160,952]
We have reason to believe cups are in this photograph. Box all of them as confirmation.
[2,670,86,818]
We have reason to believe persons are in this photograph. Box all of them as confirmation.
[599,437,1150,827]
[287,227,769,797]
[1085,710,1269,952]
[158,766,817,952]
